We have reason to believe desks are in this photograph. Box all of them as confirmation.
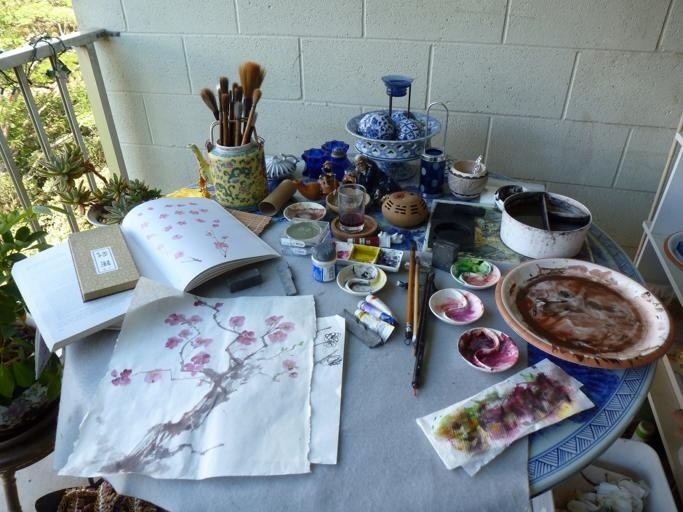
[65,172,658,500]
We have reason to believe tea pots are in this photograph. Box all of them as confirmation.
[184,118,268,210]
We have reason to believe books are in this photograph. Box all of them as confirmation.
[69,222,139,302]
[11,197,282,354]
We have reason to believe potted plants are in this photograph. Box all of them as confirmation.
[31,135,165,226]
[0,204,62,469]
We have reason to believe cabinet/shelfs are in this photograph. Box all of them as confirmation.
[634,113,682,504]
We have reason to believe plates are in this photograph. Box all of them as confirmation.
[428,257,520,373]
[333,241,405,296]
[282,189,379,239]
[494,257,675,372]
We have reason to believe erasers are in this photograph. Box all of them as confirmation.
[228,268,262,293]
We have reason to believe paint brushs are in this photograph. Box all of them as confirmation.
[405,238,436,396]
[201,63,265,146]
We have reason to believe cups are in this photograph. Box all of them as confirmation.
[447,159,488,201]
[309,254,336,283]
[420,148,448,199]
[337,183,365,234]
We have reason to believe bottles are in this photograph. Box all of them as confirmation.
[299,148,324,197]
[321,140,351,173]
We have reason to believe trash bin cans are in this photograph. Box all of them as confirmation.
[531,437,679,512]
